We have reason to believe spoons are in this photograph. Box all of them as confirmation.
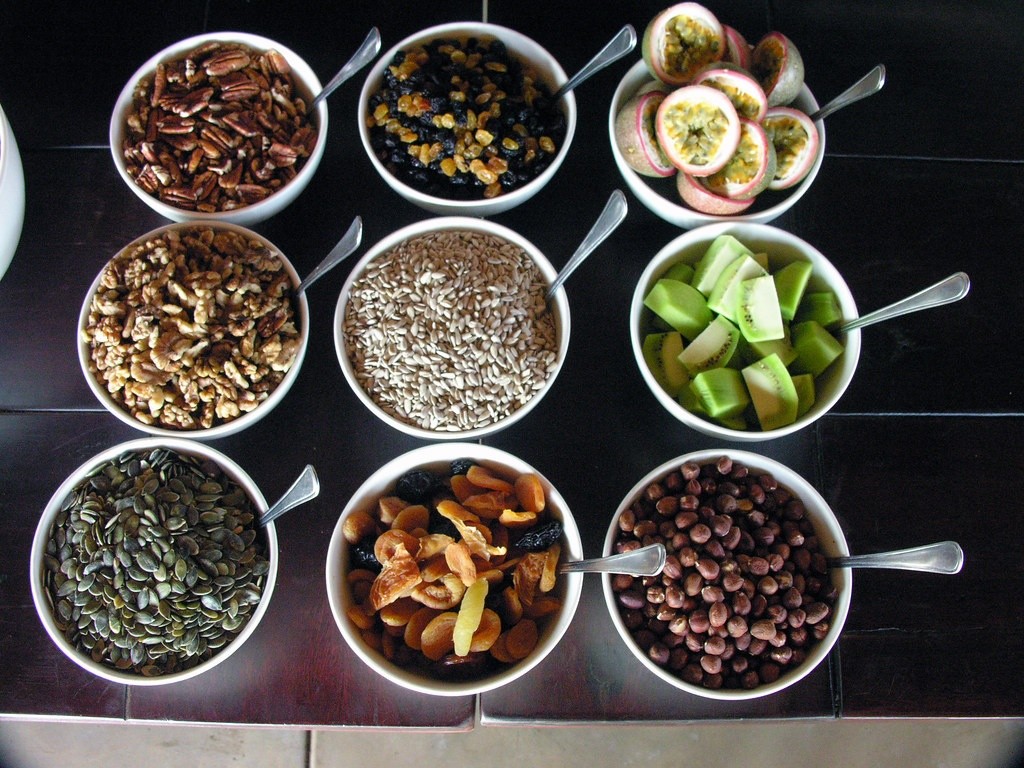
[326,442,584,697]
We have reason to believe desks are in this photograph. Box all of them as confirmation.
[0,0,1024,732]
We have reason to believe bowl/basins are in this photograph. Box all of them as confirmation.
[609,45,826,230]
[109,31,328,228]
[30,436,279,686]
[77,220,309,440]
[630,222,862,442]
[333,215,571,440]
[358,21,578,217]
[601,449,853,700]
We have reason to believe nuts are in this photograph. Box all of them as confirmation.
[344,231,561,432]
[40,449,272,678]
[119,41,318,213]
[79,222,305,429]
[613,456,839,691]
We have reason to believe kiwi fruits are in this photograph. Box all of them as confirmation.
[646,235,844,433]
